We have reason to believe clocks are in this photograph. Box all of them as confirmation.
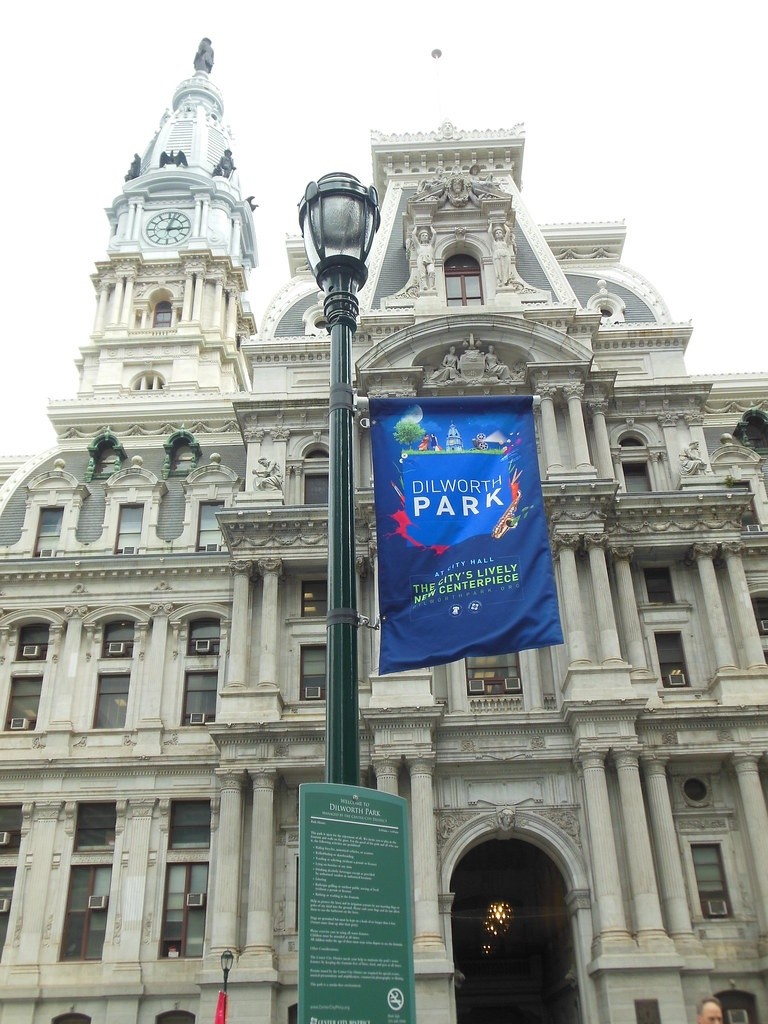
[147,211,191,246]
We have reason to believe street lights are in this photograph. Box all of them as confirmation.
[221,947,234,1024]
[296,170,381,787]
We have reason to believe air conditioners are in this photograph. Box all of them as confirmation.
[0,899,10,912]
[702,899,728,919]
[305,687,322,701]
[745,525,761,532]
[88,895,109,910]
[0,832,11,846]
[10,717,30,730]
[107,642,128,657]
[195,640,213,655]
[758,620,768,635]
[665,674,685,687]
[206,543,220,551]
[189,713,208,725]
[186,892,206,907]
[503,677,522,694]
[122,546,137,554]
[467,680,485,695]
[727,1008,749,1024]
[39,548,54,558]
[22,645,41,659]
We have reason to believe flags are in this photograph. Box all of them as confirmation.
[214,991,229,1023]
[366,395,569,675]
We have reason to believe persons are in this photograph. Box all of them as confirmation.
[410,225,437,290]
[484,344,511,381]
[441,345,460,381]
[694,998,725,1023]
[487,223,511,287]
[219,146,237,179]
